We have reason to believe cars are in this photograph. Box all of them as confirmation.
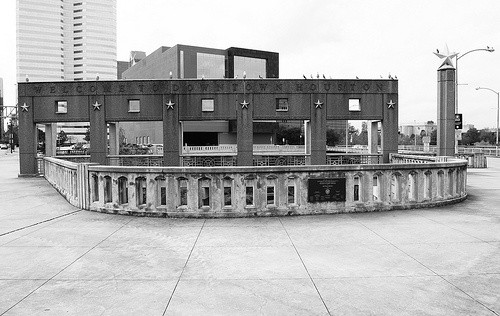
[0,143,11,150]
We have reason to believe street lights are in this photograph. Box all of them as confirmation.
[475,86,500,158]
[454,46,495,145]
